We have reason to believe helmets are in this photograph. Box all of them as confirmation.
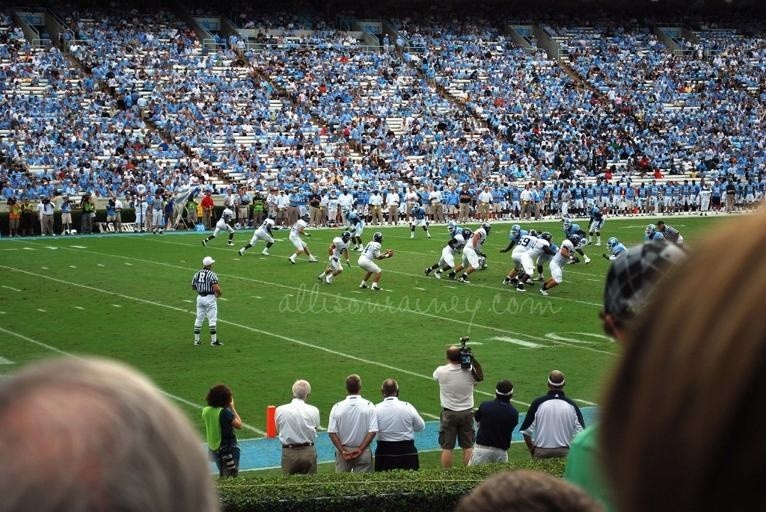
[342,232,350,242]
[447,221,491,240]
[301,212,310,221]
[511,219,581,247]
[608,237,617,248]
[373,232,382,242]
[645,224,656,236]
[269,213,276,220]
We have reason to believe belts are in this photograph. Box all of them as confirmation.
[283,443,314,448]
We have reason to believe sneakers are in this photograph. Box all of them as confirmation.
[211,341,223,346]
[503,276,549,295]
[318,275,332,284]
[424,267,470,283]
[194,340,205,345]
[585,258,591,264]
[238,249,243,256]
[359,283,383,290]
[309,257,317,262]
[351,246,364,251]
[262,251,269,255]
[289,257,295,264]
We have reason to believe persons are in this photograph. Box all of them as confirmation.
[191,256,225,347]
[273,379,320,475]
[590,204,765,511]
[374,378,427,471]
[465,379,519,465]
[1,0,764,292]
[519,369,587,460]
[432,346,478,468]
[559,234,691,511]
[454,469,607,510]
[202,384,243,478]
[0,354,222,510]
[326,372,379,472]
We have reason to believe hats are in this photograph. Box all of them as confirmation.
[203,256,215,266]
[605,239,686,334]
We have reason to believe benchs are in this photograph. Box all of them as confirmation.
[1,12,766,219]
